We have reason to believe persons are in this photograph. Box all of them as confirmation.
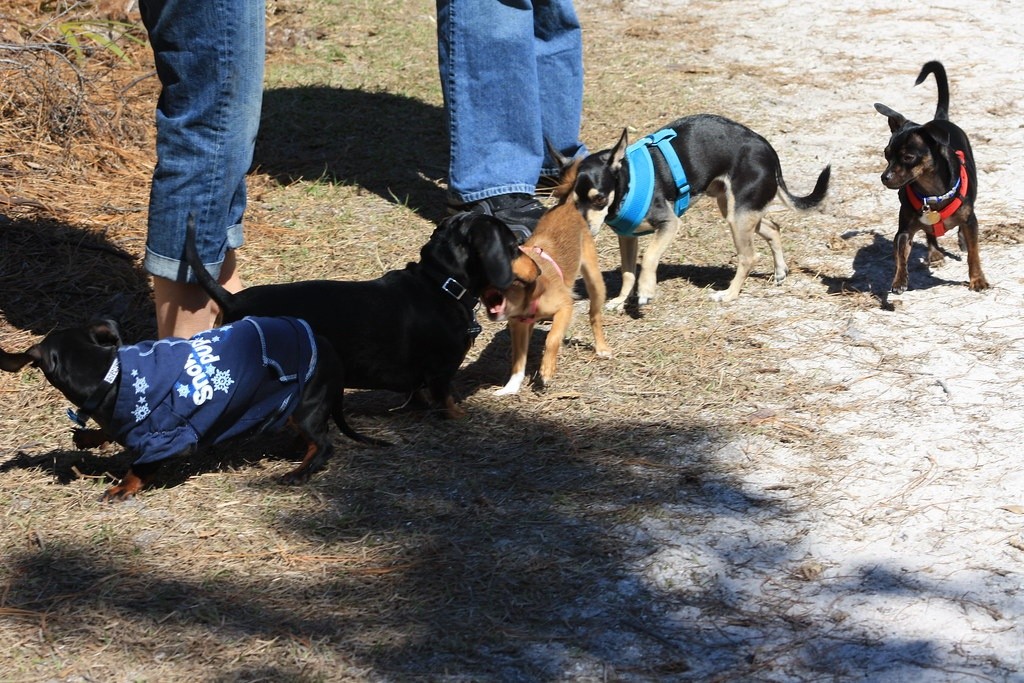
[138,0,264,341]
[438,0,584,219]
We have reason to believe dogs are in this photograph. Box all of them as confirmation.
[497,158,608,395]
[0,317,400,504]
[871,60,990,294]
[182,209,516,418]
[541,113,832,313]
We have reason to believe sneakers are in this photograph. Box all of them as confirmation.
[446,185,550,244]
[534,174,565,196]
